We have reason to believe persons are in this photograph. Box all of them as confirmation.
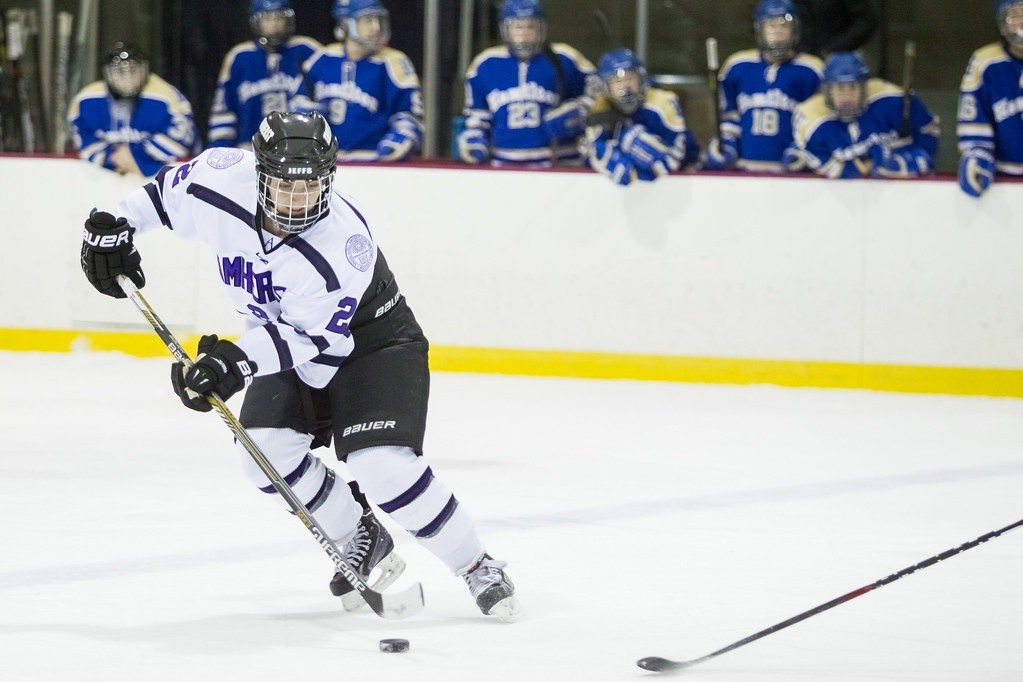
[455,0,596,168]
[290,0,424,162]
[208,0,324,150]
[706,0,825,173]
[955,0,1023,197]
[82,109,520,621]
[68,46,203,177]
[791,53,941,180]
[584,49,699,185]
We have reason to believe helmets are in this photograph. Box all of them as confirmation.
[248,0,298,54]
[336,0,391,50]
[497,0,545,58]
[597,48,648,115]
[824,51,869,121]
[251,110,338,235]
[101,43,147,100]
[753,0,800,64]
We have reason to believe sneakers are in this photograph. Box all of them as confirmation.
[330,480,404,612]
[462,550,519,623]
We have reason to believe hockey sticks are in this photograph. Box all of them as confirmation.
[5,1,100,154]
[114,272,444,619]
[705,34,723,155]
[638,517,1023,675]
[902,37,917,137]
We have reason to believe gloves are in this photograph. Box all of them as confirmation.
[80,207,146,299]
[873,150,920,179]
[830,155,877,181]
[781,143,807,174]
[170,334,254,412]
[459,128,488,165]
[704,135,736,169]
[958,148,993,196]
[375,129,414,161]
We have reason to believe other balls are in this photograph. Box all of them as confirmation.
[379,638,410,653]
